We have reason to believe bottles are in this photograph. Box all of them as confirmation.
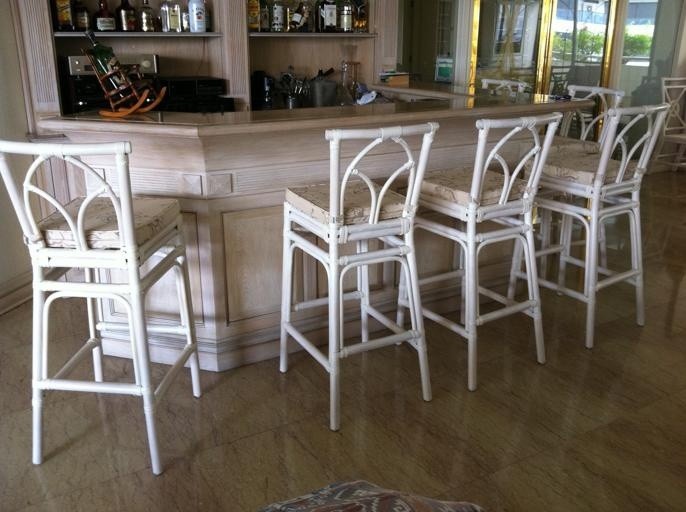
[55,0,211,33]
[248,0,368,34]
[336,62,353,106]
[85,29,137,108]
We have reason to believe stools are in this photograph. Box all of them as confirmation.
[507,102,672,350]
[280,120,442,433]
[480,78,528,94]
[534,85,627,285]
[393,110,563,393]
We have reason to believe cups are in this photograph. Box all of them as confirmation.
[251,76,276,109]
[285,95,304,109]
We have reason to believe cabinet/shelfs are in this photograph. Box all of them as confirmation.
[44,0,378,115]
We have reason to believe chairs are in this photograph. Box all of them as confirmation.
[0,141,203,478]
[644,76,686,175]
